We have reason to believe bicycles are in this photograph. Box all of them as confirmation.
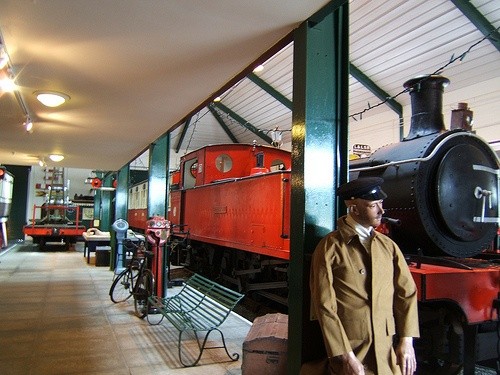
[108,232,156,319]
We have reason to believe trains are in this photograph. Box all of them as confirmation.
[112,72,500,375]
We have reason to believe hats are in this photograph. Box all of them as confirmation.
[335,178,386,200]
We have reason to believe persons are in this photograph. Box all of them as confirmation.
[310,177,420,375]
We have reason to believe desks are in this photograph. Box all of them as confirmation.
[83,231,145,264]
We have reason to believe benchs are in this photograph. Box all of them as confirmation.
[146,273,245,367]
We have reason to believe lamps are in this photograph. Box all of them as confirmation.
[22,114,33,132]
[49,154,64,162]
[32,90,71,108]
